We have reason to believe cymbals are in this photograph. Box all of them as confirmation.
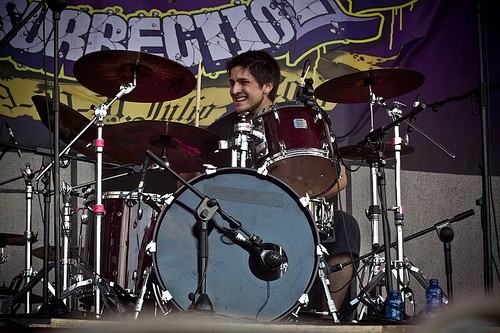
[315,69,426,103]
[31,94,98,160]
[338,143,415,161]
[73,49,196,103]
[103,120,220,174]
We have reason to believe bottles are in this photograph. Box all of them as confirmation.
[385,290,403,322]
[425,279,443,319]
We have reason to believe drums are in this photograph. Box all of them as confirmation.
[246,100,341,199]
[303,199,335,240]
[151,166,321,324]
[81,192,170,317]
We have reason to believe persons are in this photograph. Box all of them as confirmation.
[174,49,362,323]
